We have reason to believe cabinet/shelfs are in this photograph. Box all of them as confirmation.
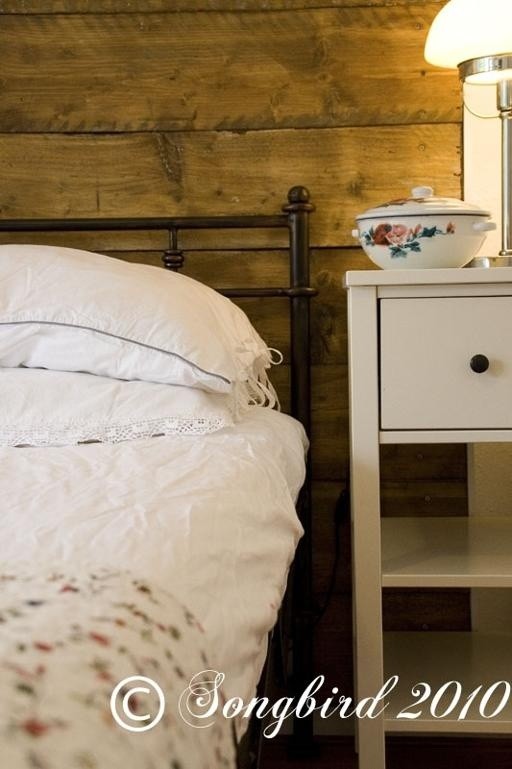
[343,266,512,769]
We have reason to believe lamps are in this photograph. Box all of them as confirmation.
[423,0,512,268]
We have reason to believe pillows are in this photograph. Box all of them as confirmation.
[0,365,253,447]
[0,241,272,391]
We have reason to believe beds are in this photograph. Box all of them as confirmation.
[0,185,316,769]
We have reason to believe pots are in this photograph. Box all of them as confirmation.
[350,181,498,270]
